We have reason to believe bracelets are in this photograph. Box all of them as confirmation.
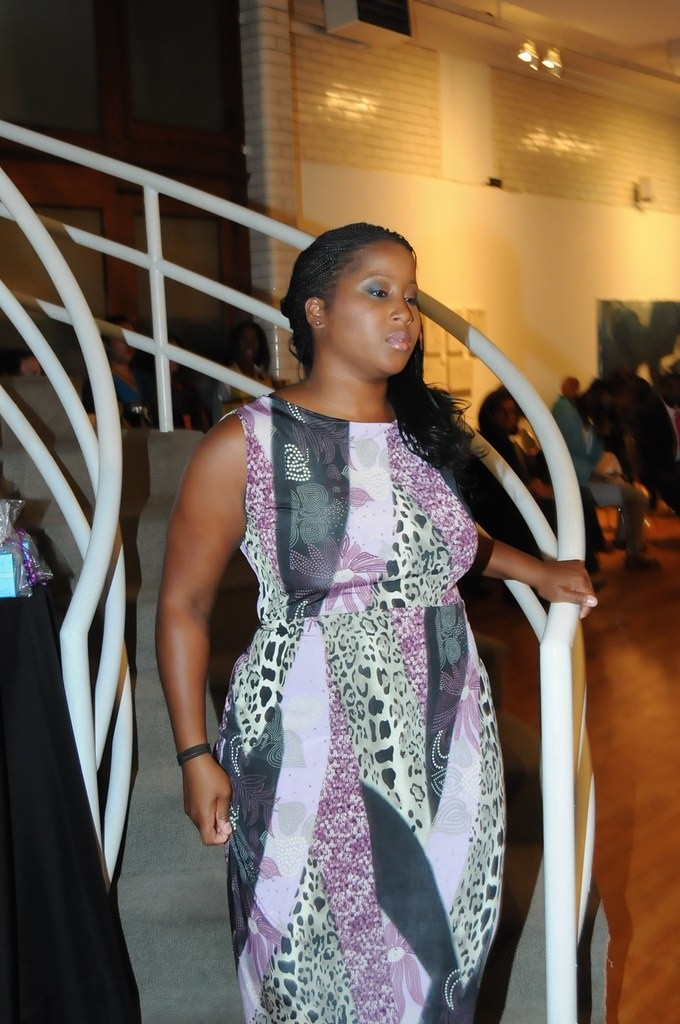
[178,742,211,765]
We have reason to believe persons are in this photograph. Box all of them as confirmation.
[152,224,599,1023]
[16,315,274,432]
[478,376,680,589]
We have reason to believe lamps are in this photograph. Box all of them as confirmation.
[516,38,538,71]
[540,45,562,78]
